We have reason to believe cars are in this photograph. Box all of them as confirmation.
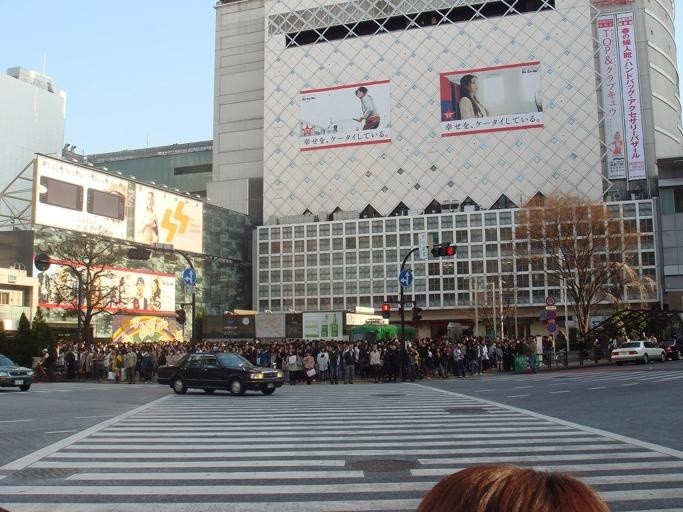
[157,352,285,395]
[611,339,683,366]
[0,353,35,390]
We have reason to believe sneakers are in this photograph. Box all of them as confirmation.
[289,378,353,385]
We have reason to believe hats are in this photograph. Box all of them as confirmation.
[42,349,48,352]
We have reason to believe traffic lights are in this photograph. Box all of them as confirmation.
[176,309,185,324]
[127,249,151,260]
[412,305,422,324]
[431,243,457,258]
[382,303,390,319]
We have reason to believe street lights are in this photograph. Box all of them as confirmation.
[481,279,506,340]
[564,277,576,353]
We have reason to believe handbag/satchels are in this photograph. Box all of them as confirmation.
[307,368,316,377]
[107,371,116,379]
[289,355,296,364]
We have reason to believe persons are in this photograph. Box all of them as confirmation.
[118,276,129,308]
[354,86,379,131]
[458,74,488,120]
[141,192,160,244]
[34,330,682,386]
[414,464,608,512]
[133,278,148,310]
[150,279,163,310]
